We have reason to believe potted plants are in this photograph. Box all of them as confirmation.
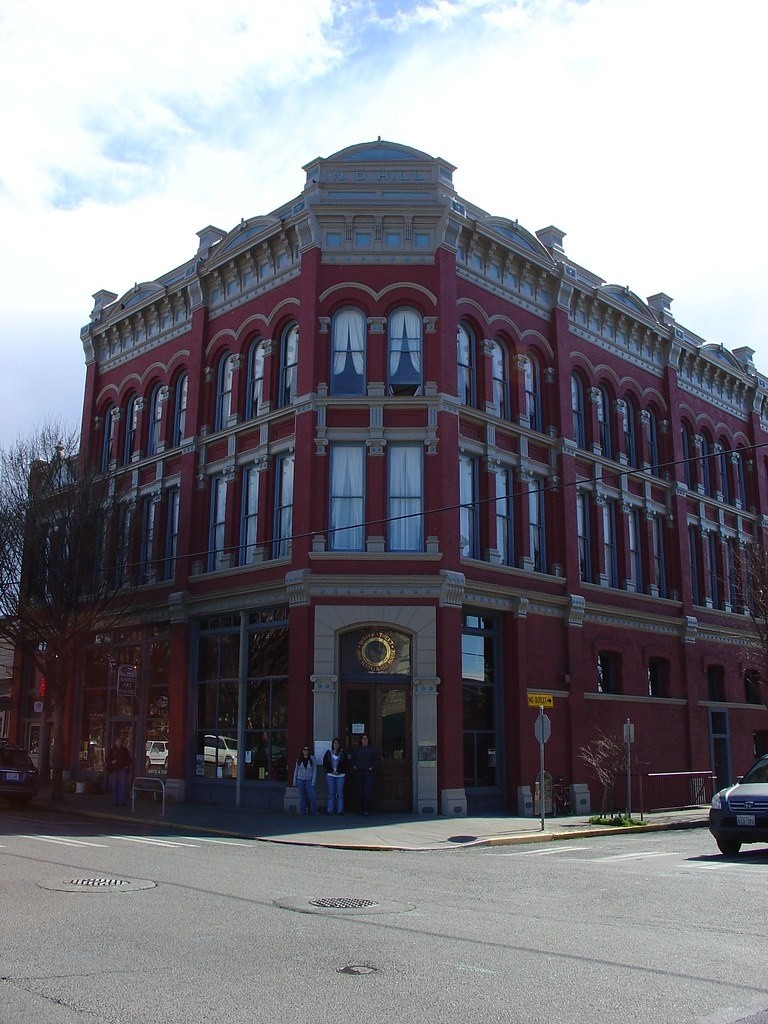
[73,772,94,794]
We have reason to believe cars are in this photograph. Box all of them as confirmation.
[709,754,768,855]
[204,735,237,765]
[145,741,169,766]
[0,736,41,804]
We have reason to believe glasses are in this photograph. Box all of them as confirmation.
[304,749,309,751]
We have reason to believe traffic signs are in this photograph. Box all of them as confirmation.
[527,693,553,708]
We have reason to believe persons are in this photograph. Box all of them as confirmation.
[109,737,130,806]
[353,733,383,817]
[292,745,320,817]
[323,738,349,815]
